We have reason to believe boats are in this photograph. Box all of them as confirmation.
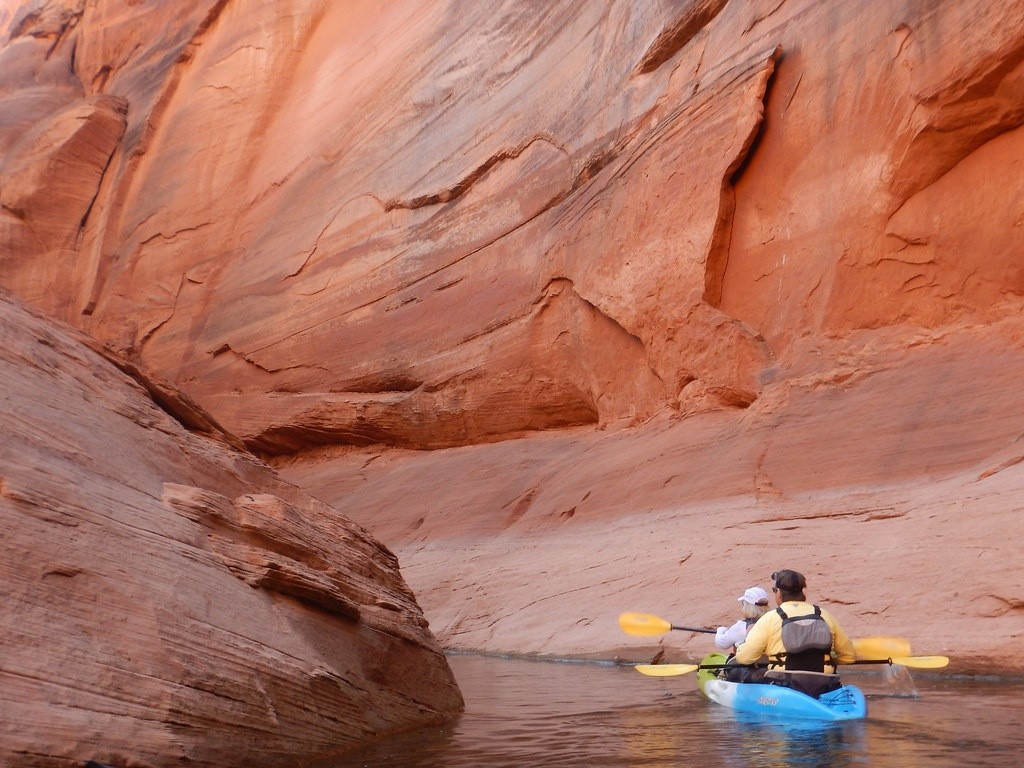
[696,651,867,723]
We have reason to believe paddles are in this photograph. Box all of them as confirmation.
[634,656,949,677]
[619,611,912,661]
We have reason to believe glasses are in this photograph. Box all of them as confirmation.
[772,587,778,594]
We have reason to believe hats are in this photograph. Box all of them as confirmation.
[771,570,807,591]
[738,586,769,606]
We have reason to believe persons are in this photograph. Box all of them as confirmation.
[714,569,857,683]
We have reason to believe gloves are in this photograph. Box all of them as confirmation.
[717,627,728,634]
